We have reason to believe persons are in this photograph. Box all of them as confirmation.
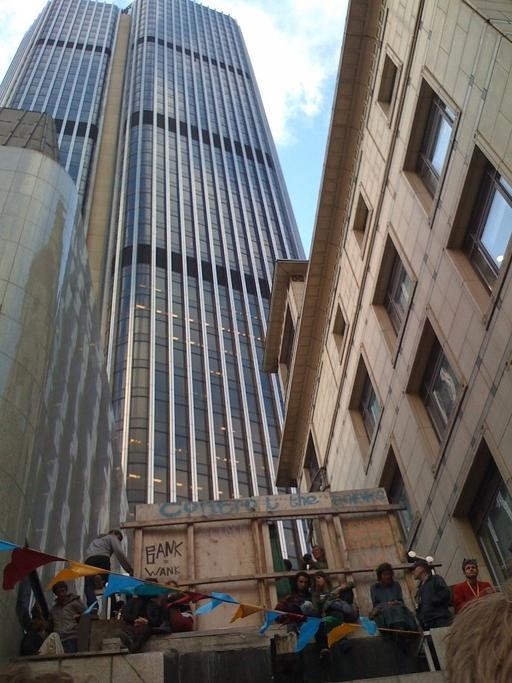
[444,592,511,683]
[19,530,193,655]
[276,545,512,657]
